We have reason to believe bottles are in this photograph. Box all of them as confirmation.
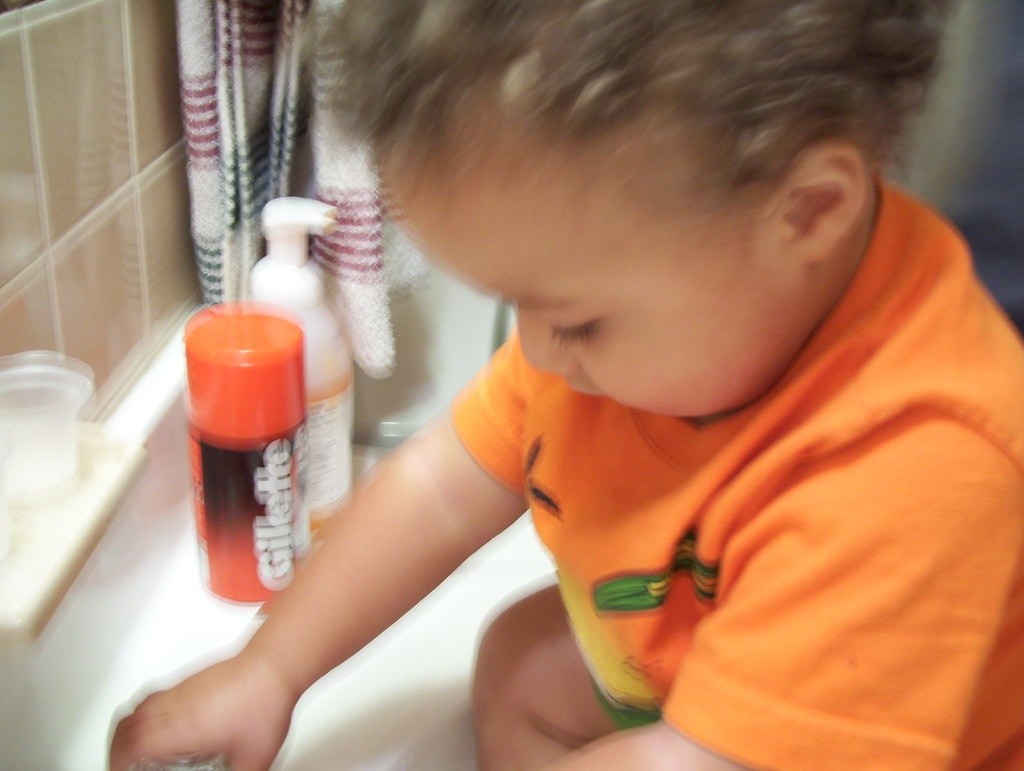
[184,304,310,609]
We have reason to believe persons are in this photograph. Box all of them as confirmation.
[110,0,1024,771]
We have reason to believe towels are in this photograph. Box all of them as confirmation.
[170,1,426,381]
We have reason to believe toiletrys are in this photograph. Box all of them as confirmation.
[244,194,359,540]
[182,300,304,601]
